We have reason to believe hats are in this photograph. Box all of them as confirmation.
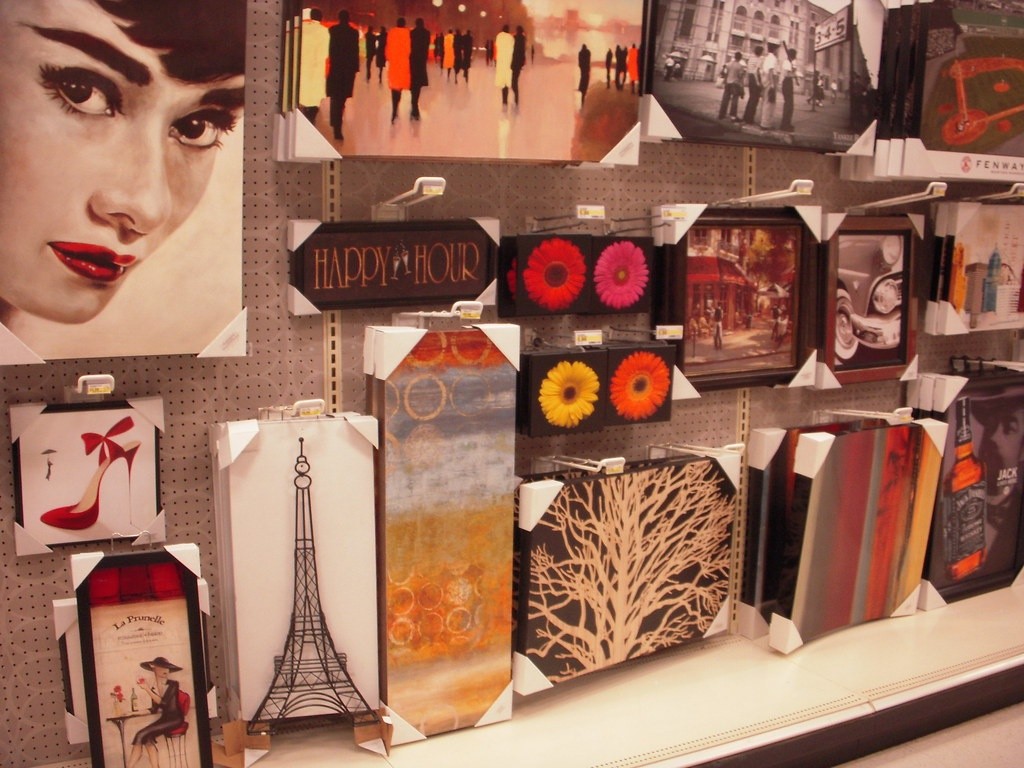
[969,386,1024,424]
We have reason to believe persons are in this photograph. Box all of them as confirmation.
[433,27,473,84]
[969,386,1023,579]
[324,9,360,141]
[688,300,724,351]
[409,18,430,121]
[831,80,838,103]
[733,306,752,328]
[383,17,411,125]
[816,74,825,106]
[301,9,329,123]
[768,282,787,338]
[606,48,613,90]
[530,44,535,65]
[485,39,497,67]
[719,43,798,131]
[2,0,247,330]
[578,44,592,111]
[665,56,680,82]
[511,25,527,106]
[495,25,515,104]
[365,26,388,83]
[615,43,639,95]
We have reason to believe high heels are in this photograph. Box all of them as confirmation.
[40,417,143,530]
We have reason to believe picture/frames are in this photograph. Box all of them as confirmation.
[907,377,1022,613]
[287,216,502,316]
[67,543,214,768]
[656,202,920,400]
[10,397,164,557]
[52,579,217,745]
